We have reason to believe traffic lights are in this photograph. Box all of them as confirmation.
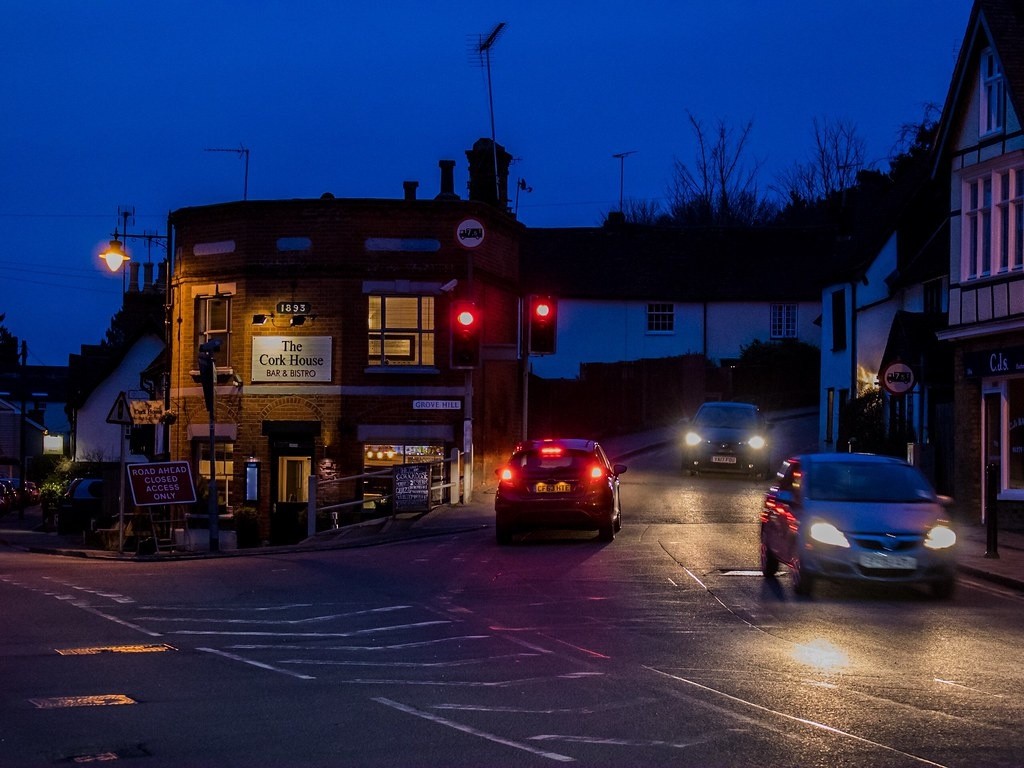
[448,299,483,370]
[527,294,560,355]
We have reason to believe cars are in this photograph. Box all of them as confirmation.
[55,476,110,535]
[676,402,774,482]
[757,451,959,603]
[0,478,41,517]
[494,437,628,547]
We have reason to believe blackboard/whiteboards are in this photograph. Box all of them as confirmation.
[393,462,432,511]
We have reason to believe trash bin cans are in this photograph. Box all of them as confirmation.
[73,479,103,534]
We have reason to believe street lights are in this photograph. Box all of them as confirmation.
[98,207,173,462]
